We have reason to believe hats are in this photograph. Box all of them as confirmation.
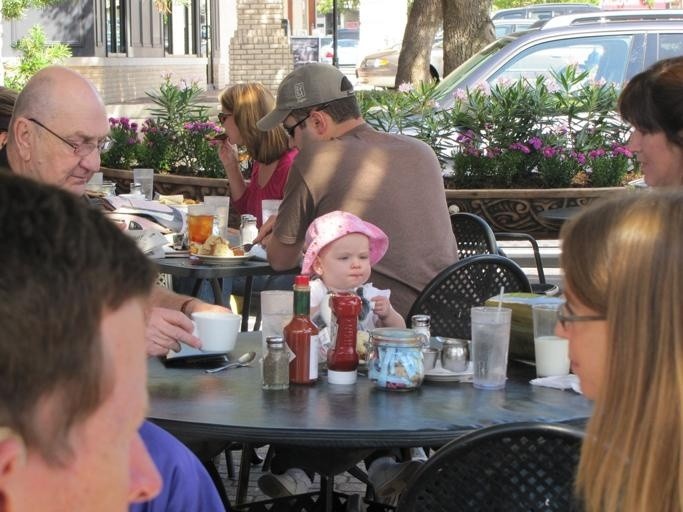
[256,63,354,130]
[301,212,389,274]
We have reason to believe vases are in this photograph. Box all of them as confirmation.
[446,188,633,239]
[99,167,249,203]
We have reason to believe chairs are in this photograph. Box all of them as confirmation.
[395,420,624,510]
[402,253,532,338]
[448,213,558,298]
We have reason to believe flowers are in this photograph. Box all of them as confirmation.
[106,118,229,175]
[453,129,635,187]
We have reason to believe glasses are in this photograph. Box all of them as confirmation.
[218,113,233,125]
[555,302,608,330]
[27,119,115,156]
[283,103,329,137]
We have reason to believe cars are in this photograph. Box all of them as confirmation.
[323,39,361,66]
[355,19,537,89]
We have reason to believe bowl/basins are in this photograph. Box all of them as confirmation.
[119,194,146,201]
[442,339,472,371]
[421,349,437,371]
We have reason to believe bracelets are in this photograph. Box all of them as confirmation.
[180,296,196,313]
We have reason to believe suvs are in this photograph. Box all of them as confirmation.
[493,4,602,20]
[362,20,681,186]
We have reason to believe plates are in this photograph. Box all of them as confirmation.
[191,248,256,267]
[414,357,481,382]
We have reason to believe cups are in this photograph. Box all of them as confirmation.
[260,291,293,362]
[130,183,144,195]
[102,182,118,200]
[262,199,286,228]
[134,169,154,201]
[87,173,102,190]
[469,310,512,389]
[204,197,229,241]
[191,313,240,352]
[186,205,214,247]
[531,305,572,381]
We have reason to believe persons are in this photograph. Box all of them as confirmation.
[128,421,226,512]
[0,171,161,512]
[617,57,683,186]
[555,188,682,512]
[217,81,302,232]
[0,65,234,357]
[253,61,458,323]
[300,210,407,361]
[0,86,20,170]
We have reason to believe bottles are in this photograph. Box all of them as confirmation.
[365,328,428,392]
[128,183,142,195]
[327,293,362,384]
[283,275,320,385]
[239,214,253,246]
[242,217,258,246]
[262,337,288,391]
[412,315,431,348]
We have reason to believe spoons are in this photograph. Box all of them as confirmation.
[205,349,256,373]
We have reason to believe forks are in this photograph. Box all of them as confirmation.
[230,232,265,254]
